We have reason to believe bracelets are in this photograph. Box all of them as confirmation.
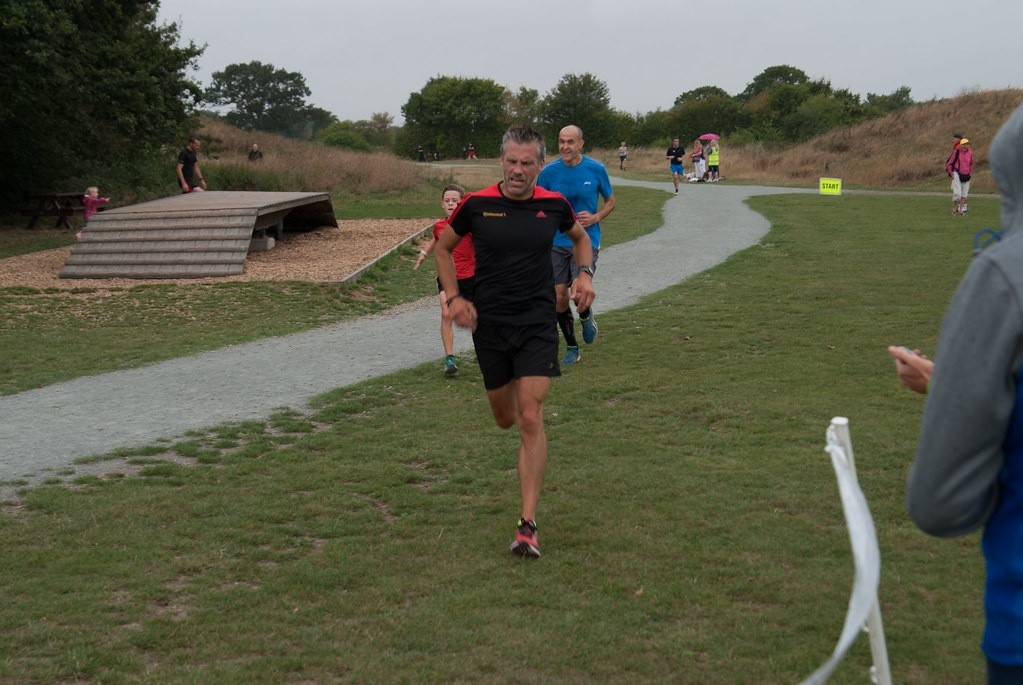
[200,178,204,180]
[421,250,428,257]
[445,293,462,308]
[578,264,594,278]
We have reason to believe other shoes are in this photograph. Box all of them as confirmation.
[957,204,967,211]
[952,210,956,215]
[675,189,678,194]
[961,212,968,216]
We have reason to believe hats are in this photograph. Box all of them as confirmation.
[960,138,970,145]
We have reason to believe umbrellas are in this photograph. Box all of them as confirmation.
[699,133,720,140]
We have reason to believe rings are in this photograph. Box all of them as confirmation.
[582,222,583,225]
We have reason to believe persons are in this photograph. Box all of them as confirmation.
[414,184,476,377]
[435,124,595,559]
[619,141,629,170]
[417,145,428,163]
[462,143,478,161]
[886,101,1023,685]
[75,186,111,241]
[946,134,972,217]
[536,125,616,365]
[665,136,687,195]
[689,139,720,182]
[248,143,263,163]
[177,136,208,195]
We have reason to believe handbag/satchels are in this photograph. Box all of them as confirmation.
[955,168,970,182]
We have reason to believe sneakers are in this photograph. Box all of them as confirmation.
[580,307,597,343]
[445,357,459,376]
[510,516,541,559]
[561,346,581,364]
